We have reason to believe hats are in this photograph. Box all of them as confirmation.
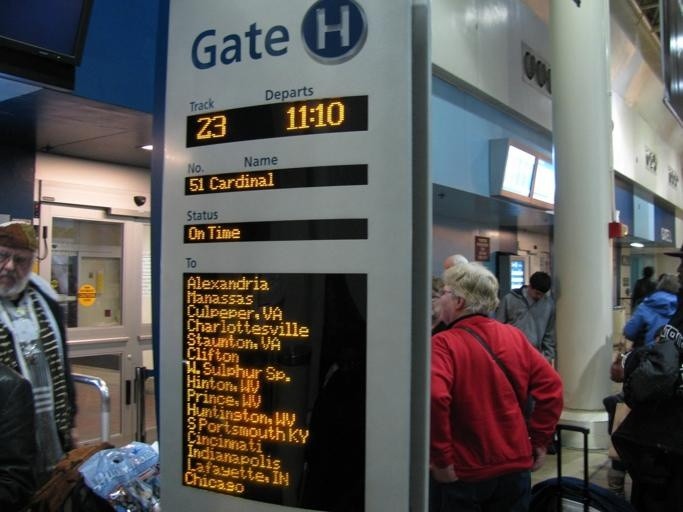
[662,242,683,260]
[0,220,39,252]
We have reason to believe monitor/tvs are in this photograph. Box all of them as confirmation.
[498,255,529,293]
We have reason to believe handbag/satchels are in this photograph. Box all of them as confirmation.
[611,335,631,383]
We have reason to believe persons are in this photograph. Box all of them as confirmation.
[491,271,559,457]
[440,254,468,276]
[603,243,683,512]
[0,360,39,511]
[424,260,566,510]
[0,220,82,485]
